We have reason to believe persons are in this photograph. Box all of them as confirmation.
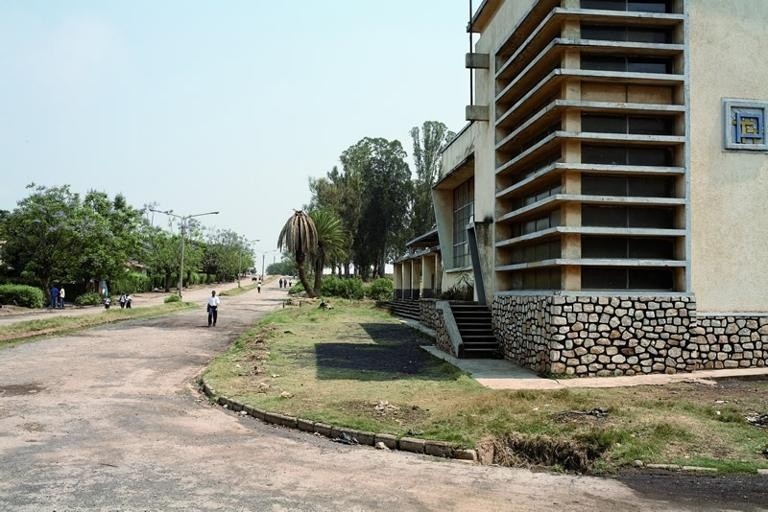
[103,290,134,311]
[206,290,220,327]
[257,278,262,294]
[279,277,292,288]
[50,285,67,309]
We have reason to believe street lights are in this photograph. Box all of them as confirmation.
[224,239,261,288]
[262,248,279,283]
[148,208,220,302]
